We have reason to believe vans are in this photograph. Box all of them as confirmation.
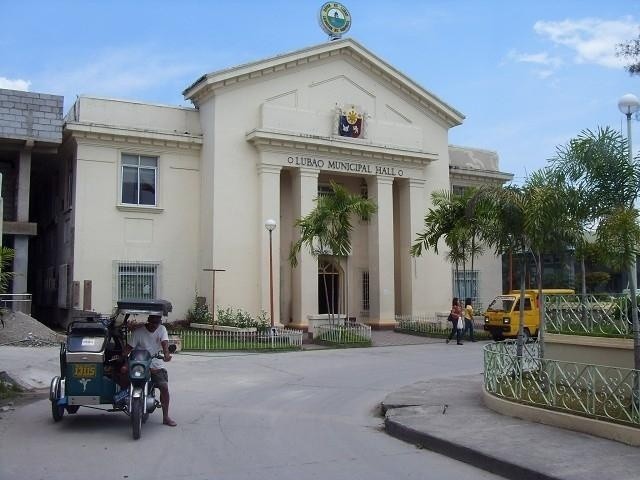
[481,289,575,342]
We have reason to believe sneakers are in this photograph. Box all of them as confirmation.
[456,342,463,345]
[162,418,177,428]
[471,340,478,343]
[446,339,450,344]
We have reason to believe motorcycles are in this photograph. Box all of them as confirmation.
[47,295,181,439]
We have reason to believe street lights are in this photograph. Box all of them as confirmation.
[617,94,640,296]
[264,220,279,330]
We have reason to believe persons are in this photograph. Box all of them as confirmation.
[116,315,178,427]
[446,298,464,345]
[460,297,478,343]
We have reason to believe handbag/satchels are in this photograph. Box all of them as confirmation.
[447,310,453,322]
[456,316,464,330]
